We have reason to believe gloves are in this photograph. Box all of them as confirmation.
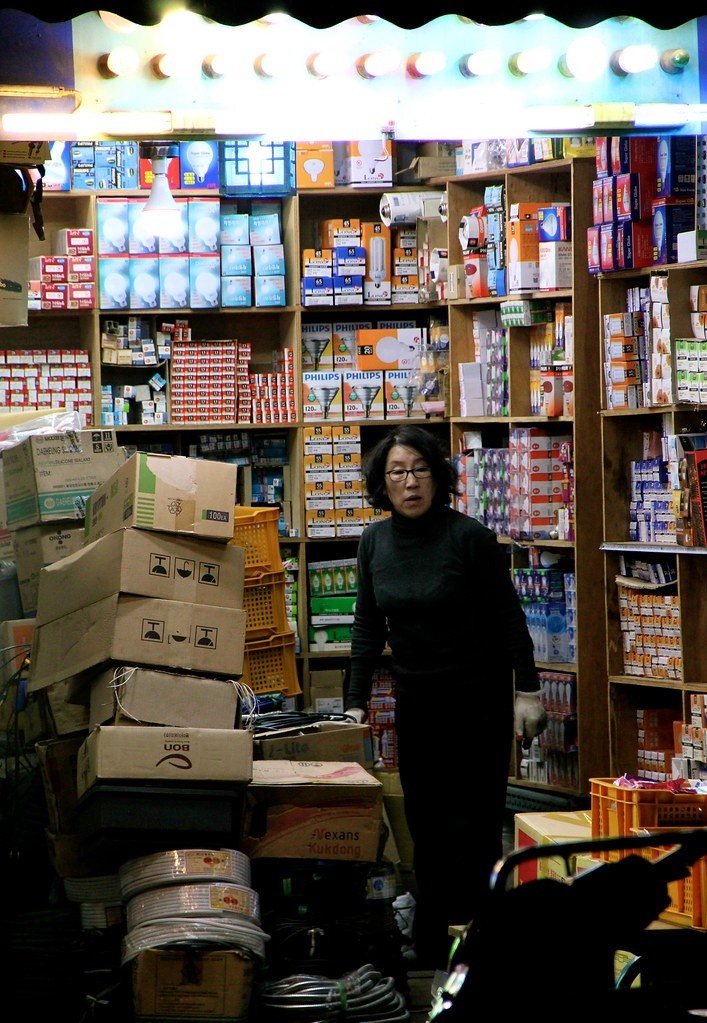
[341,708,365,724]
[514,688,548,750]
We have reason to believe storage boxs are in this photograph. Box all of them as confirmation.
[1,143,706,1021]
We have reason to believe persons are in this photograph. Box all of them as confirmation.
[343,423,548,972]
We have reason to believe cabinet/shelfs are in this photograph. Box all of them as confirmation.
[30,190,448,714]
[432,155,602,794]
[590,261,706,777]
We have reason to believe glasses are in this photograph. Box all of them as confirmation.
[384,465,435,482]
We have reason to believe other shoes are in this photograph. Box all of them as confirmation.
[400,948,447,970]
